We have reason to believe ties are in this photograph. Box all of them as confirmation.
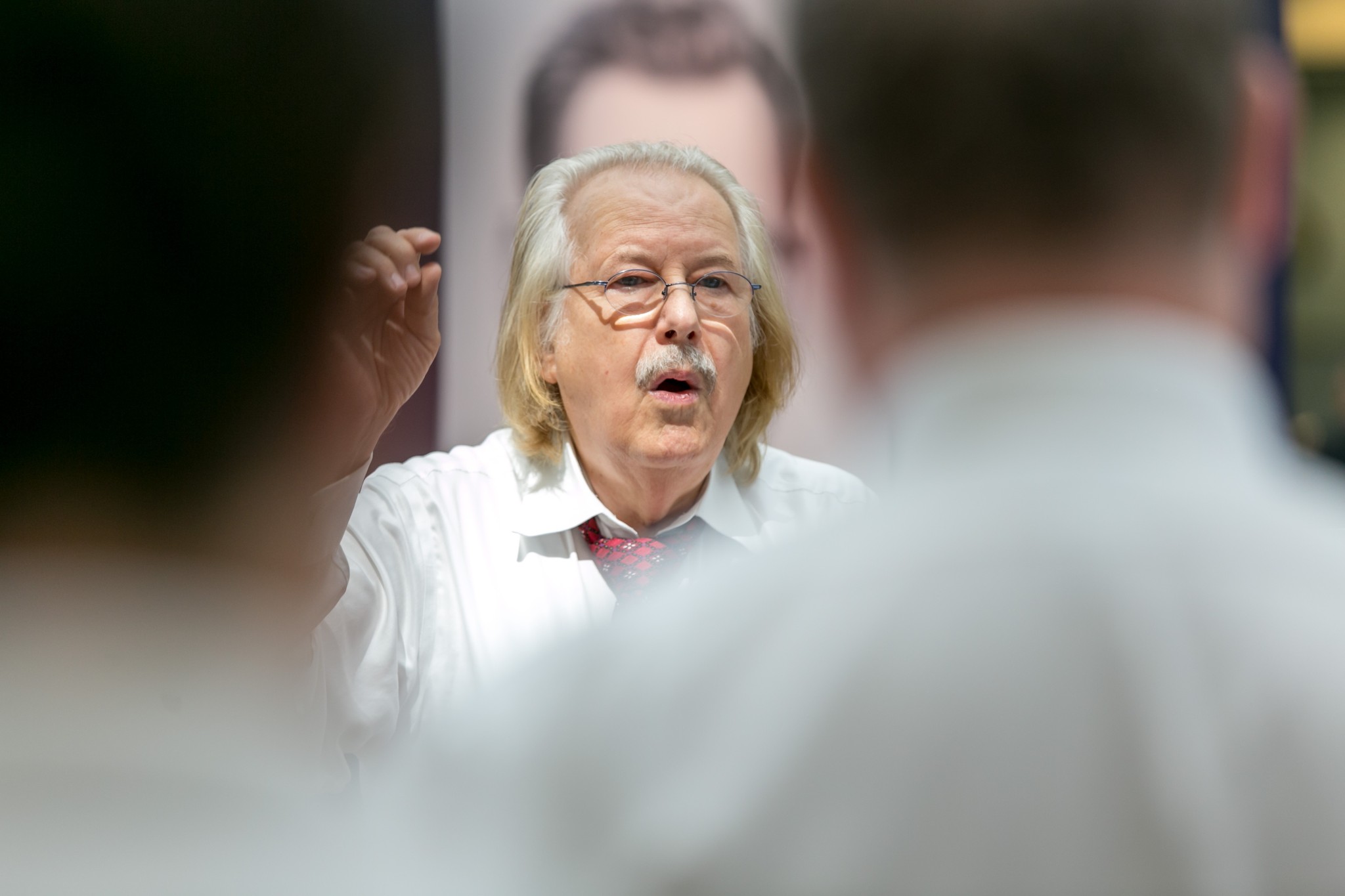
[579,514,710,611]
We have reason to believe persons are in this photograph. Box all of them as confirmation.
[1,0,1344,896]
[290,141,874,752]
[522,1,806,269]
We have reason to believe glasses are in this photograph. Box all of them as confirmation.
[550,269,762,321]
